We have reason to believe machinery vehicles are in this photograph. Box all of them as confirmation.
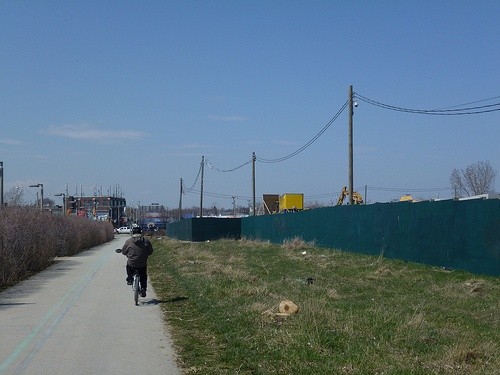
[336,185,363,206]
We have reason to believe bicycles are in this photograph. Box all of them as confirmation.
[115,248,144,306]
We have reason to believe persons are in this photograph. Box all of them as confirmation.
[122,227,153,297]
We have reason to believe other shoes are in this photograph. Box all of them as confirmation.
[127,282,132,285]
[140,290,146,297]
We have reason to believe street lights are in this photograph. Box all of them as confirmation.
[54,193,65,215]
[28,183,44,212]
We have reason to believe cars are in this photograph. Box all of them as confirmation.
[114,221,143,235]
